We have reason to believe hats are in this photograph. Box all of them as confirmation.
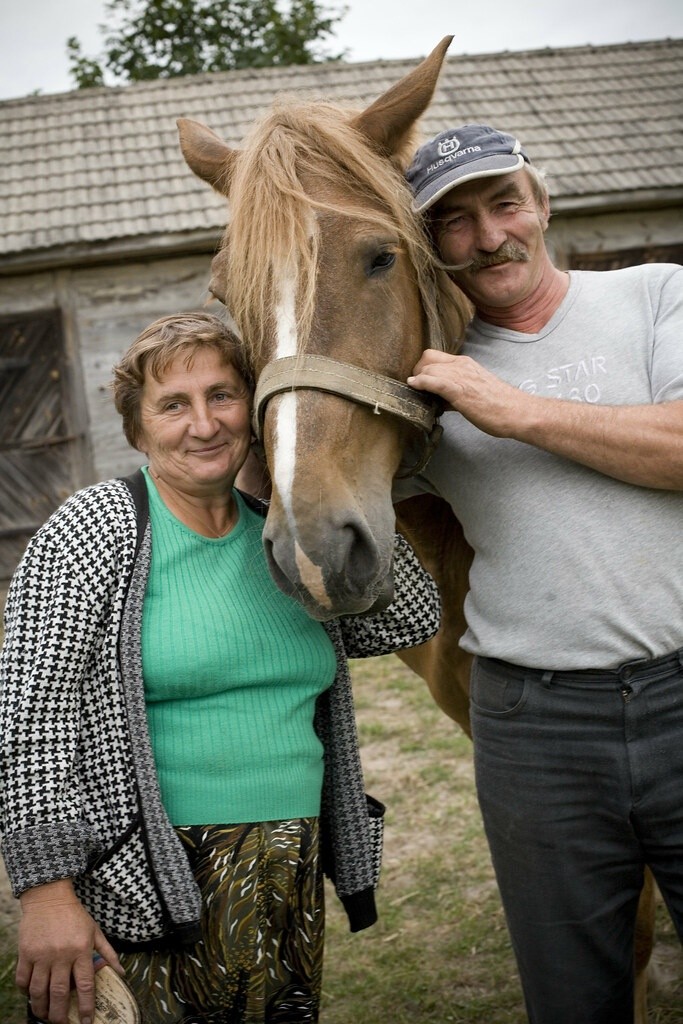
[405,125,530,216]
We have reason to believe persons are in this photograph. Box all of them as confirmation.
[0,314,440,1024]
[408,125,683,1024]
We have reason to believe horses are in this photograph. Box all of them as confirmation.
[174,33,656,1024]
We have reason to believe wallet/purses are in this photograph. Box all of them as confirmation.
[28,950,139,1023]
